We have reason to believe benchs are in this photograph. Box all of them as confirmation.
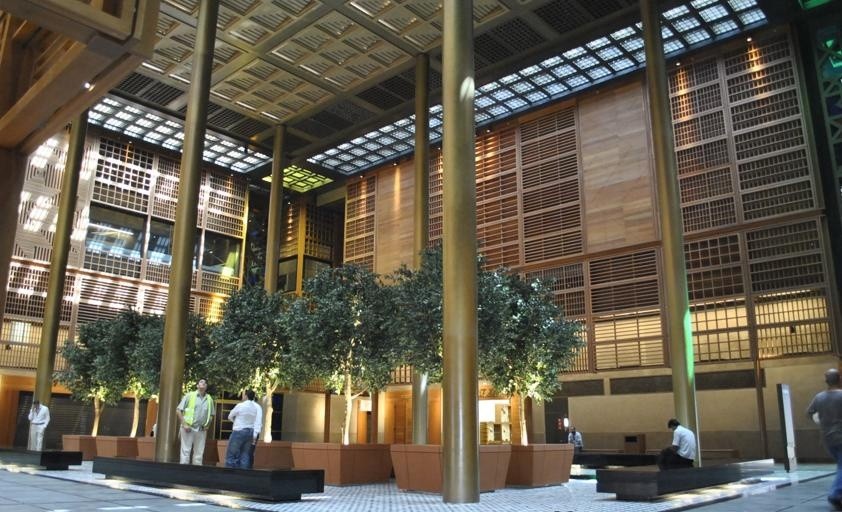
[92,454,326,502]
[571,450,775,504]
[0,448,83,472]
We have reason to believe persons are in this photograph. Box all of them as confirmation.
[176,378,215,465]
[805,368,842,511]
[568,426,583,450]
[28,400,50,450]
[225,390,262,467]
[658,419,695,468]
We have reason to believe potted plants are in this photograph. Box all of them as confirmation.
[63,316,128,461]
[372,244,512,496]
[208,286,292,469]
[137,314,207,461]
[95,312,139,459]
[478,266,575,489]
[290,265,407,487]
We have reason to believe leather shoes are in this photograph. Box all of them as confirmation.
[829,498,842,511]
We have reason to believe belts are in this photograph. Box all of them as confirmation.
[32,423,44,425]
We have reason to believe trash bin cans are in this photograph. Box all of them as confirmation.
[624,433,645,455]
